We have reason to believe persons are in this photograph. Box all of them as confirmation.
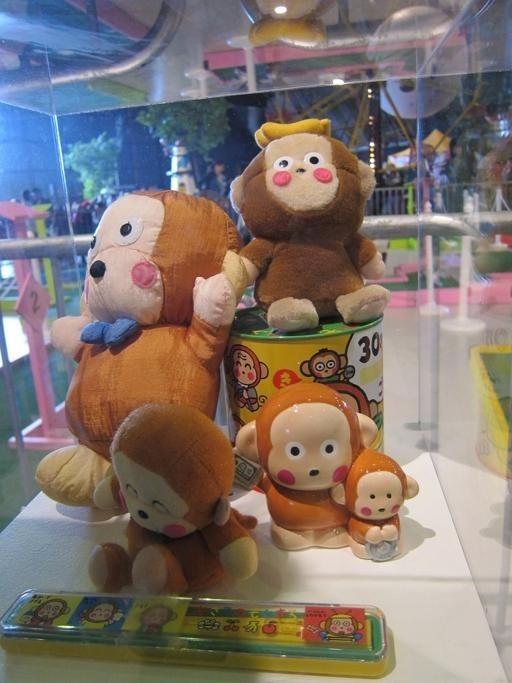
[193,135,512,225]
[0,182,170,269]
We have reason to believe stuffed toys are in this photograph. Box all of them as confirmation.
[86,400,262,596]
[33,185,245,507]
[221,118,392,332]
